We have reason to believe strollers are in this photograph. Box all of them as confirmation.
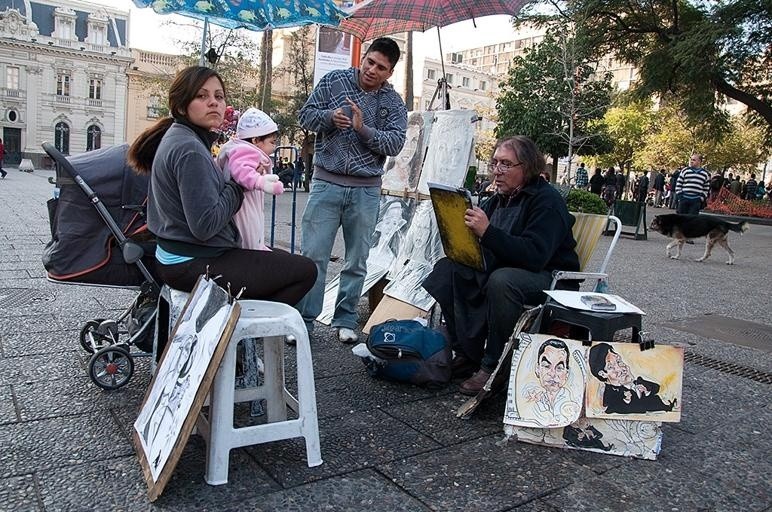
[38,143,173,390]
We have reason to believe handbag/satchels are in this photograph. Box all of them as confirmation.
[361,321,447,380]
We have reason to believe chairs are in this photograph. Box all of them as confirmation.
[433,212,622,419]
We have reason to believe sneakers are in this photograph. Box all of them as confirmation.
[339,327,359,343]
[285,334,295,344]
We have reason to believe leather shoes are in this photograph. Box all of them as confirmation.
[450,355,473,372]
[460,370,508,396]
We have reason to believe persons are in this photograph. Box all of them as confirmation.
[146,66,318,379]
[285,36,408,345]
[653,170,666,207]
[590,343,677,415]
[521,339,577,422]
[590,168,604,196]
[0,139,8,178]
[272,153,312,192]
[616,169,625,199]
[711,169,772,202]
[576,163,588,190]
[675,154,711,244]
[604,167,616,204]
[217,108,283,251]
[471,176,497,194]
[638,170,649,202]
[421,134,582,397]
[559,166,568,184]
[631,174,639,201]
[669,171,679,209]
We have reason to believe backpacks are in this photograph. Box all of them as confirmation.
[3,143,9,163]
[351,318,453,390]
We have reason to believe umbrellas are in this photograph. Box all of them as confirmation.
[133,0,348,32]
[337,1,532,109]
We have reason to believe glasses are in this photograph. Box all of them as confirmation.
[491,161,525,173]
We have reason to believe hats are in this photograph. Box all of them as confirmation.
[238,108,278,139]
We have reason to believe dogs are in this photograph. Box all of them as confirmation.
[646,214,750,266]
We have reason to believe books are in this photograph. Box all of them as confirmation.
[581,295,616,311]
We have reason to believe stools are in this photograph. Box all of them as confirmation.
[195,300,323,485]
[152,284,265,417]
[537,302,642,349]
